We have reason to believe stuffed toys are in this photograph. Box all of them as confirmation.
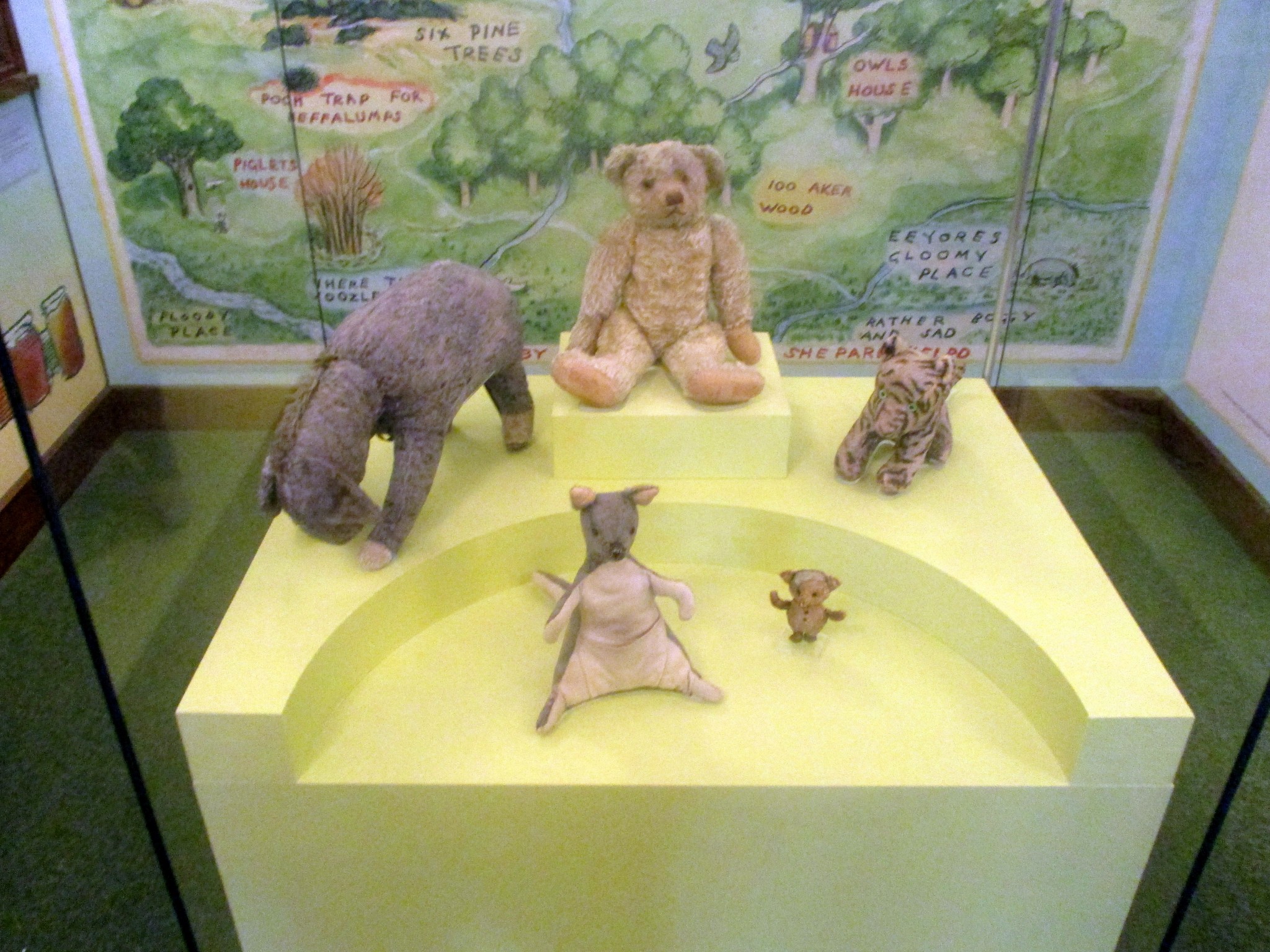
[256,260,534,570]
[552,139,764,409]
[532,485,723,733]
[834,333,965,494]
[771,569,846,642]
